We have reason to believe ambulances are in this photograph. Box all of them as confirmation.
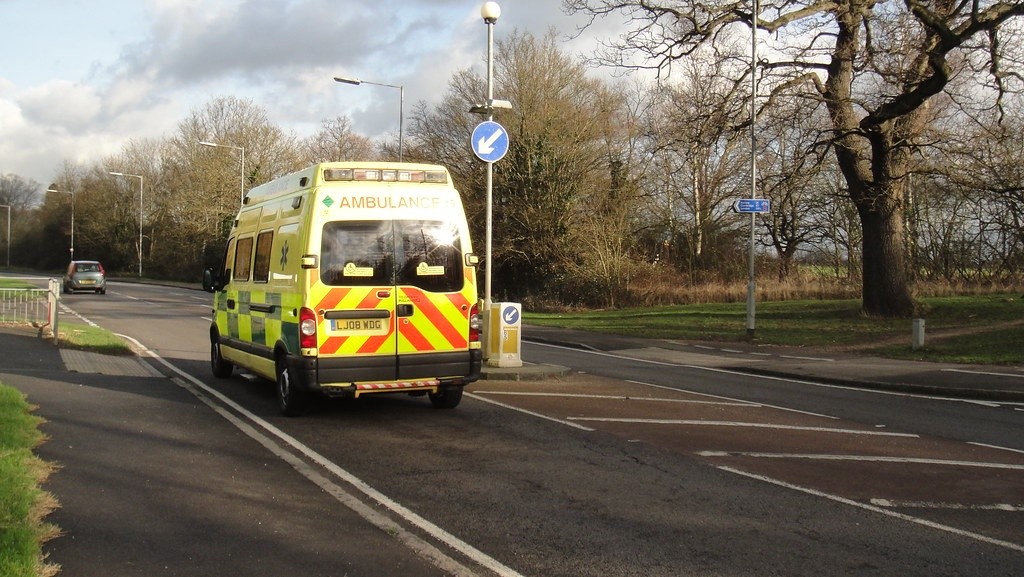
[202,161,482,418]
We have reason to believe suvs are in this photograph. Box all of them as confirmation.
[64,261,106,294]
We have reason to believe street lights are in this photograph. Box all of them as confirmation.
[200,141,245,206]
[332,75,404,161]
[46,189,75,261]
[468,2,511,358]
[109,172,142,274]
